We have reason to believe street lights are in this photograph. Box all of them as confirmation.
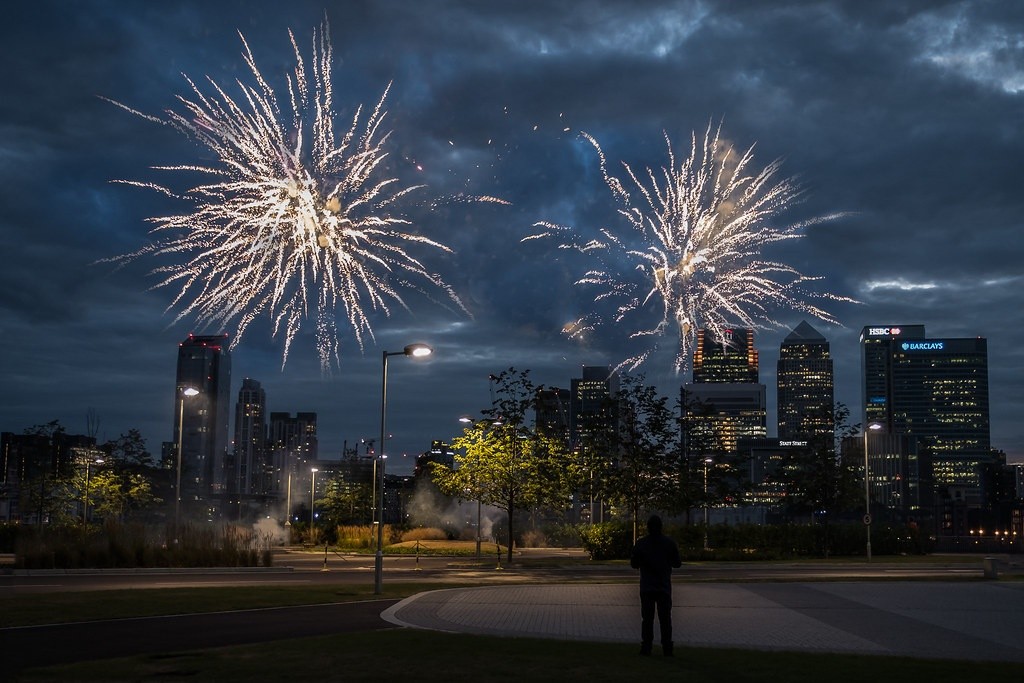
[703,456,713,547]
[864,421,884,562]
[372,454,388,526]
[459,417,481,556]
[83,459,105,532]
[310,468,319,527]
[174,381,203,539]
[374,343,433,597]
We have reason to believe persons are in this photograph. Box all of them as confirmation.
[630,515,681,656]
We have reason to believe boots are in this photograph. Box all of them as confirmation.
[662,643,674,657]
[639,641,652,657]
